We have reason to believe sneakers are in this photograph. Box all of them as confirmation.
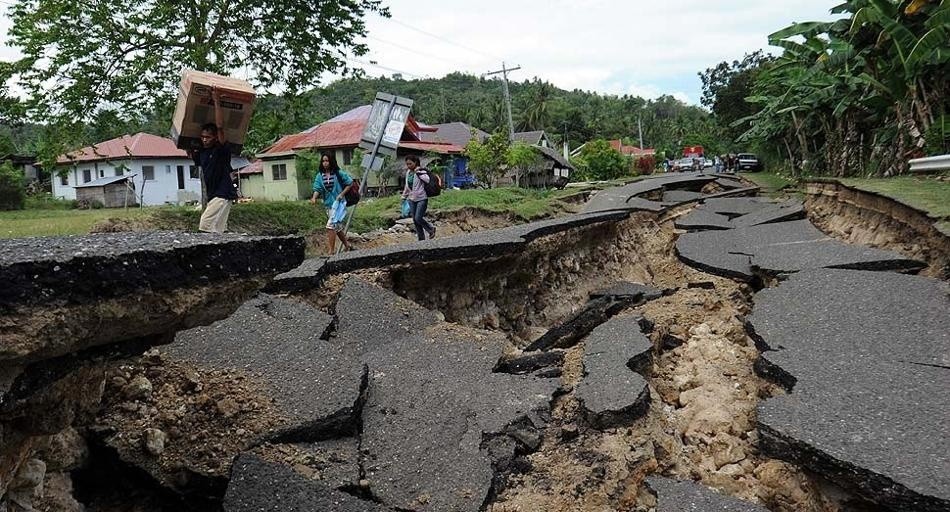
[428,227,436,239]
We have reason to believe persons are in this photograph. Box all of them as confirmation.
[186,85,237,235]
[402,155,436,243]
[691,158,703,173]
[310,151,354,258]
[715,153,733,174]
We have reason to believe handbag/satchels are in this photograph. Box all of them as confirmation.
[400,199,415,218]
[344,182,359,206]
[416,168,441,197]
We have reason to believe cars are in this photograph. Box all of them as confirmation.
[664,153,736,173]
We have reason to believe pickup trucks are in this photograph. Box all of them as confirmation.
[735,153,759,172]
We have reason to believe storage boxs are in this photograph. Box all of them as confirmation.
[168,64,259,163]
